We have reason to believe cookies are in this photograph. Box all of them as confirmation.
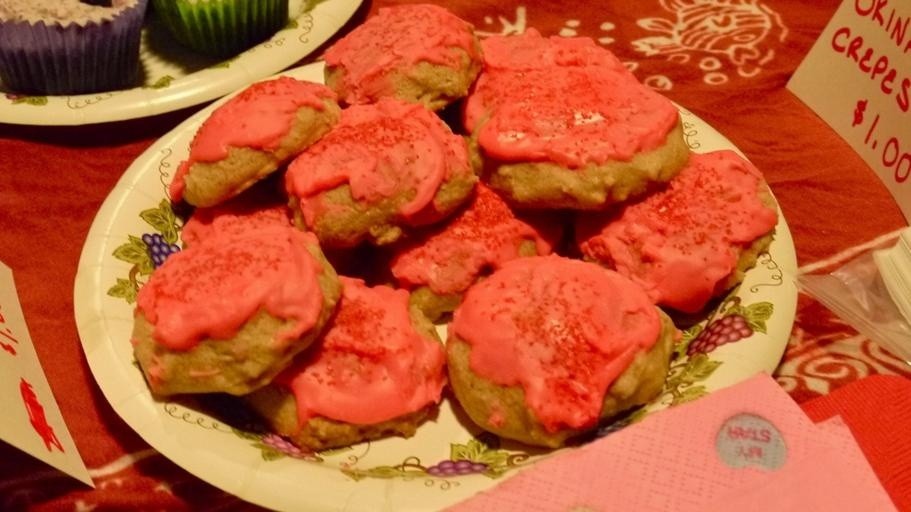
[131,1,782,452]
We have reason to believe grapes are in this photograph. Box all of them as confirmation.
[260,430,321,464]
[424,459,484,479]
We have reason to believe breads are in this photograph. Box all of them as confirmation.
[0,1,142,93]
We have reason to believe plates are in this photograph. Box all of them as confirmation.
[0,0,363,126]
[74,55,797,511]
